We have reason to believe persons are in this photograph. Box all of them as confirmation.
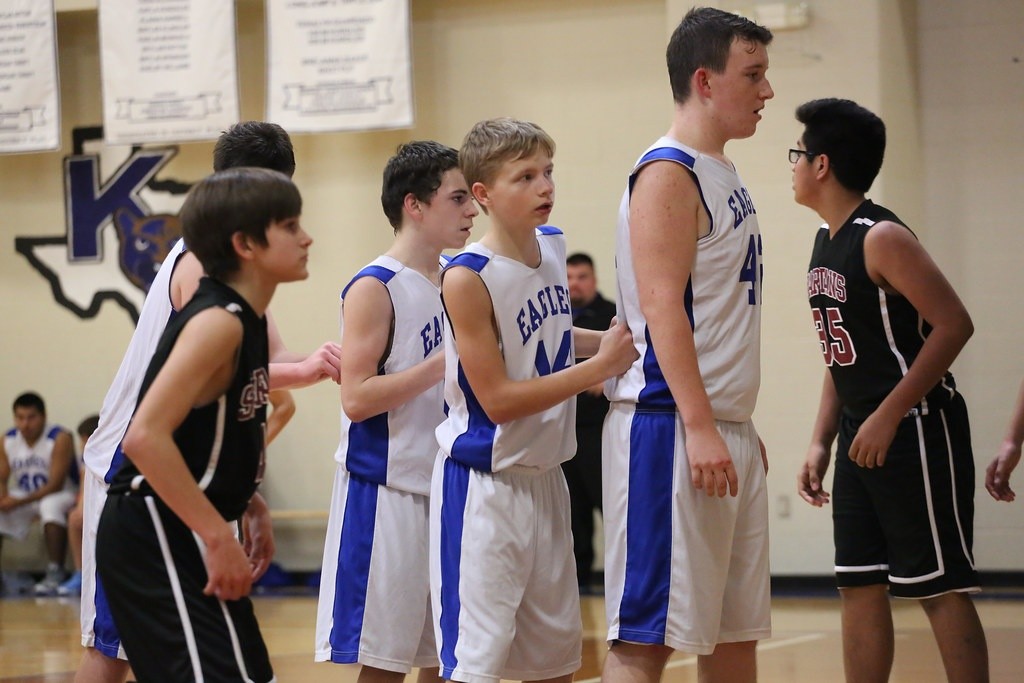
[793,99,993,683]
[316,142,479,683]
[985,375,1024,502]
[596,8,774,683]
[95,167,314,683]
[429,118,641,683]
[0,392,80,597]
[56,415,100,595]
[566,251,616,595]
[79,121,341,683]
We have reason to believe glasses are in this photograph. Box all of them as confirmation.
[789,149,818,163]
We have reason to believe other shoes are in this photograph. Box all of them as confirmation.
[56,572,81,596]
[33,570,68,594]
